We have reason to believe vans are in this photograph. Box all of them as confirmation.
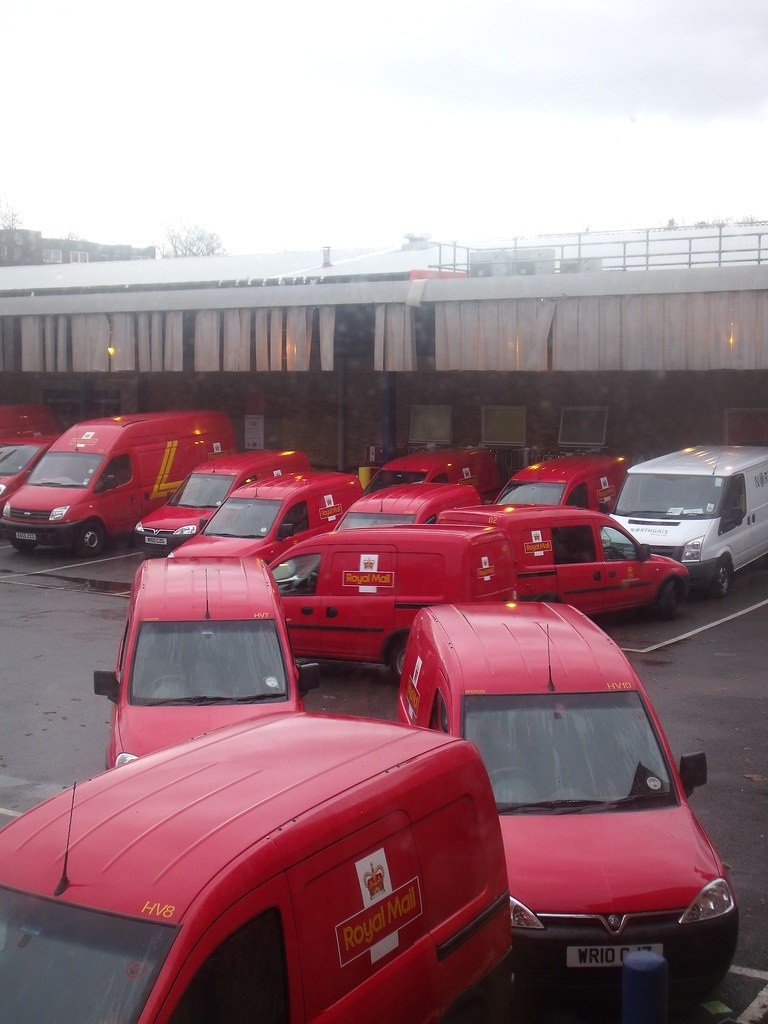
[132,449,307,554]
[330,482,482,529]
[436,505,689,624]
[363,449,503,505]
[600,444,768,597]
[398,602,738,1011]
[0,406,58,436]
[166,471,365,570]
[1,711,515,1024]
[492,456,627,517]
[0,411,240,559]
[93,554,322,781]
[267,526,519,679]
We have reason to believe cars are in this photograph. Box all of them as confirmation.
[0,435,60,512]
